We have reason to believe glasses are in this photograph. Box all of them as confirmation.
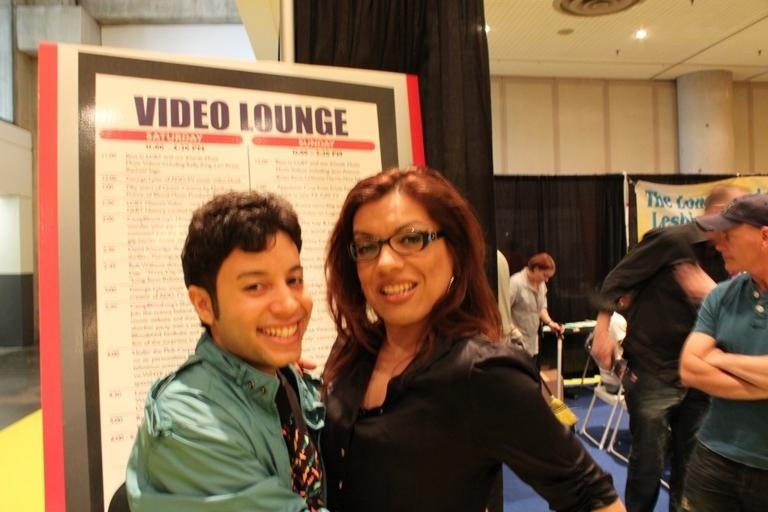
[346,226,446,263]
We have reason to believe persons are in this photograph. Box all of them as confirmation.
[588,184,749,512]
[293,163,626,512]
[590,294,627,394]
[124,189,330,512]
[508,252,564,372]
[676,194,767,512]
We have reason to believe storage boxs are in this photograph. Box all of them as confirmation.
[538,365,565,408]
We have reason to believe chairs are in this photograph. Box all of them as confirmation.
[578,345,625,450]
[606,399,631,465]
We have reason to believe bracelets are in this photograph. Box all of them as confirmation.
[546,319,552,326]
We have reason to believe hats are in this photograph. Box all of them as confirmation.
[694,190,768,234]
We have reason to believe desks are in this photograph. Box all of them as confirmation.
[538,318,600,406]
